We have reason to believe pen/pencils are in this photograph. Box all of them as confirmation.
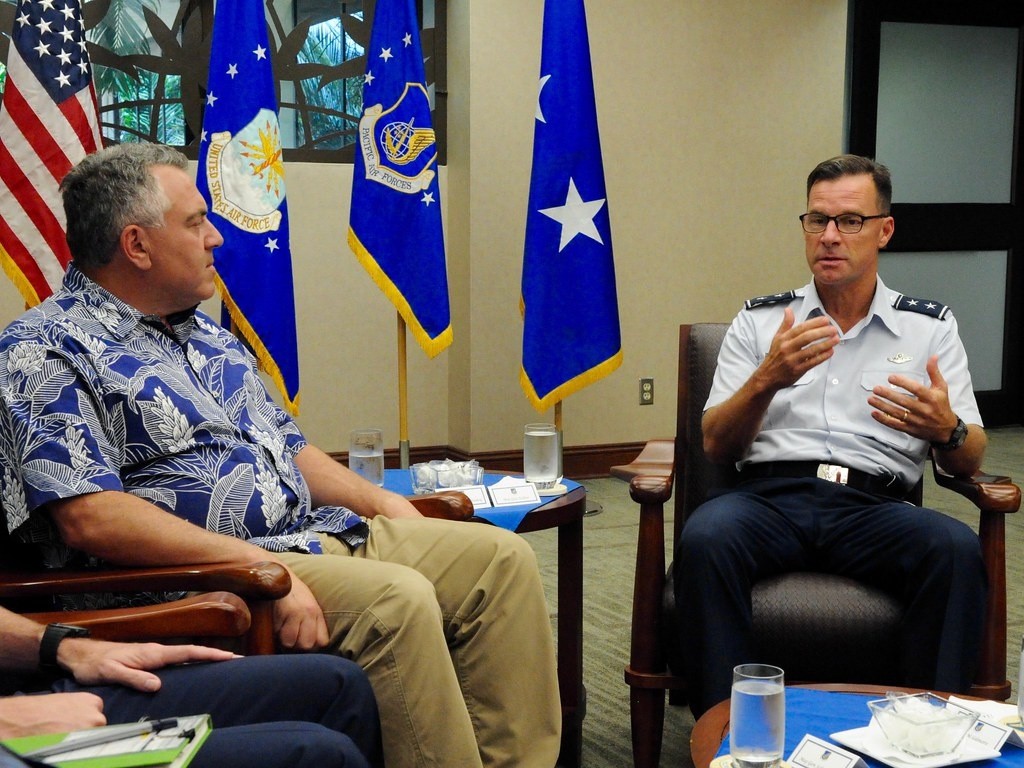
[27,715,180,762]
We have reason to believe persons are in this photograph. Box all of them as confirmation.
[0,606,385,768]
[0,142,562,768]
[674,154,989,723]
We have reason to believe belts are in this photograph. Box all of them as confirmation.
[740,457,904,500]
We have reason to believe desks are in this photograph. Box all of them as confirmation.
[689,682,1024,768]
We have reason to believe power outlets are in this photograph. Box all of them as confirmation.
[641,377,655,405]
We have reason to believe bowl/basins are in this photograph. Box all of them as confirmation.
[867,692,979,757]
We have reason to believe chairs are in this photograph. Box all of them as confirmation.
[0,492,474,654]
[16,592,251,646]
[611,323,1020,768]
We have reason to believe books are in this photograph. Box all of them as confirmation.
[0,714,212,768]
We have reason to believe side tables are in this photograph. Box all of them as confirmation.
[350,467,588,767]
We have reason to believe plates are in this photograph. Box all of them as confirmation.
[828,728,1001,767]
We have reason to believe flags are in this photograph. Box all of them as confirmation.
[519,0,624,414]
[348,0,454,359]
[195,0,300,418]
[0,0,105,328]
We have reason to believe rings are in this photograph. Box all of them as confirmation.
[901,410,908,421]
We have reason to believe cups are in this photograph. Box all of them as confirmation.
[524,423,558,489]
[349,428,384,488]
[409,460,484,494]
[729,664,785,768]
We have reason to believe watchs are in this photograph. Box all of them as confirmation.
[39,622,90,674]
[929,414,968,452]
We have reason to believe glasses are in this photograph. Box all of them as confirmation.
[799,213,887,235]
